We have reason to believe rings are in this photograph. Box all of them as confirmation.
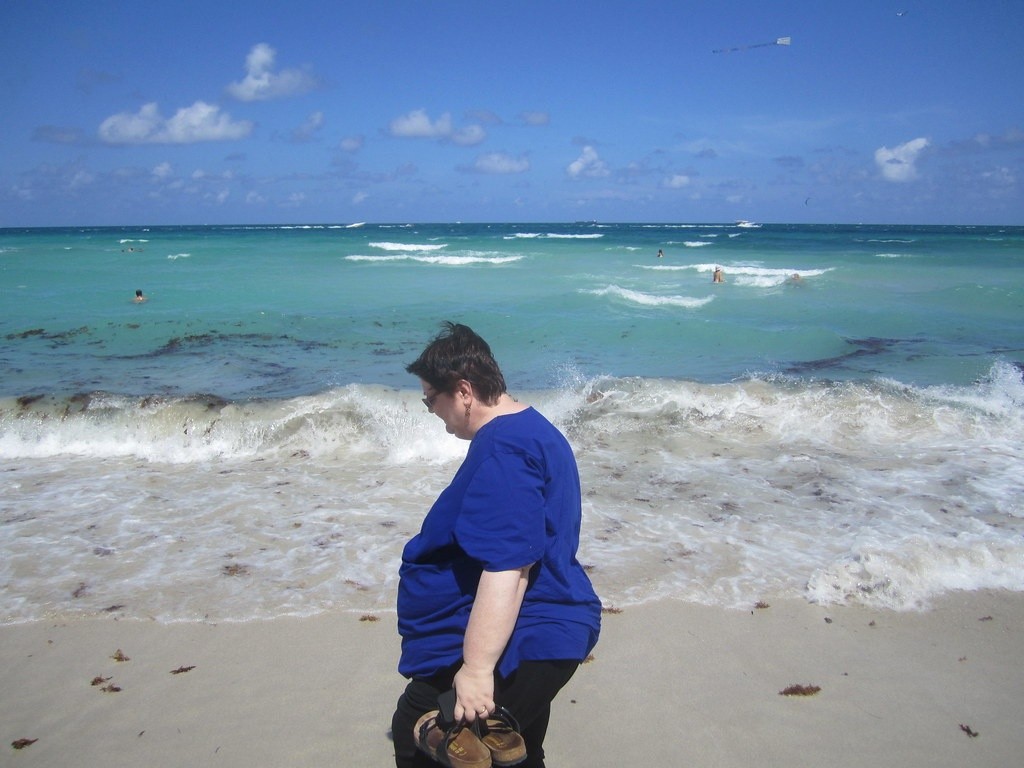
[479,708,486,715]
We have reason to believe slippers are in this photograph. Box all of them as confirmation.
[413,708,494,768]
[473,705,528,767]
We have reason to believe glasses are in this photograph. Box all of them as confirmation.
[422,389,446,408]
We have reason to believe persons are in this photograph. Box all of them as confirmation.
[658,245,799,282]
[391,319,602,768]
[121,246,145,302]
